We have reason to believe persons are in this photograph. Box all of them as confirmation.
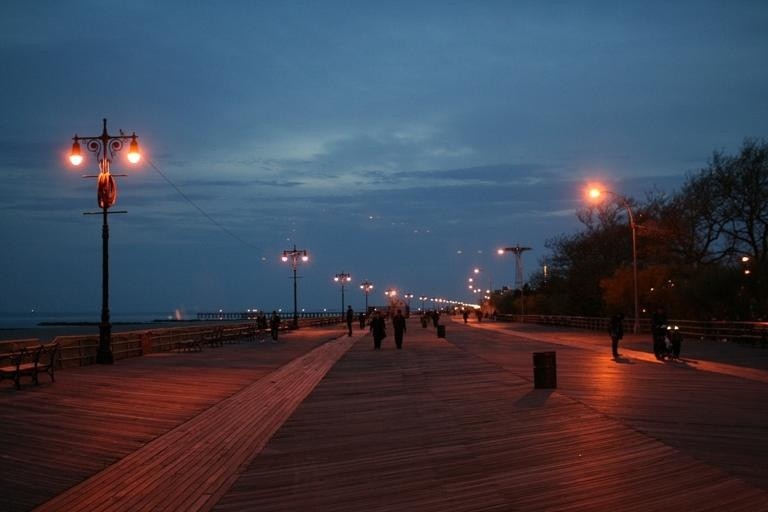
[343,304,485,352]
[268,310,282,344]
[606,311,626,357]
[650,303,668,363]
[256,310,269,344]
[670,329,684,359]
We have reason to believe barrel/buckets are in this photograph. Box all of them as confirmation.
[533,351,557,390]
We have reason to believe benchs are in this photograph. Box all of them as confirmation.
[0,342,58,387]
[174,315,354,353]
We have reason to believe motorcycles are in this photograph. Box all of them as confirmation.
[661,322,682,362]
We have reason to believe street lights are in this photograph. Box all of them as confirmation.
[68,117,142,362]
[385,288,396,301]
[334,271,352,321]
[588,188,641,336]
[360,279,374,315]
[495,243,534,321]
[281,243,309,327]
[468,268,493,321]
[405,293,464,315]
